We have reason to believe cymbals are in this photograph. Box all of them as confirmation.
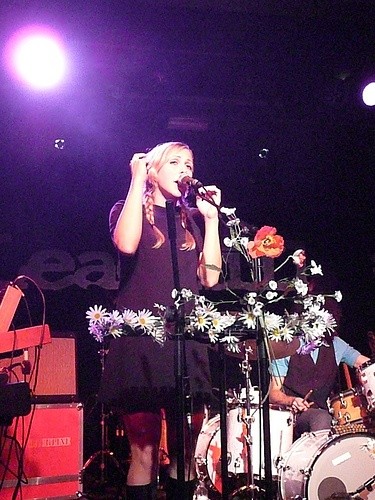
[206,334,301,360]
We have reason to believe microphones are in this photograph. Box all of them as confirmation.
[22,348,31,374]
[179,175,203,187]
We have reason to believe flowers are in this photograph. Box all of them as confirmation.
[86,206,342,355]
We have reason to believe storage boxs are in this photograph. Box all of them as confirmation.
[0,403,83,500]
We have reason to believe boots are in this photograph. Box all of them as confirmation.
[166,476,198,500]
[125,481,155,500]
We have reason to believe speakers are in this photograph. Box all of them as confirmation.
[0,403,83,500]
[0,332,79,405]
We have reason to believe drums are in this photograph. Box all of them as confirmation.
[326,385,373,427]
[355,357,375,416]
[193,405,294,500]
[275,428,375,500]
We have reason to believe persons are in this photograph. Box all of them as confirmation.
[268,331,373,439]
[107,141,223,500]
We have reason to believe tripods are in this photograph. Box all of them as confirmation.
[230,349,266,500]
[82,403,127,498]
